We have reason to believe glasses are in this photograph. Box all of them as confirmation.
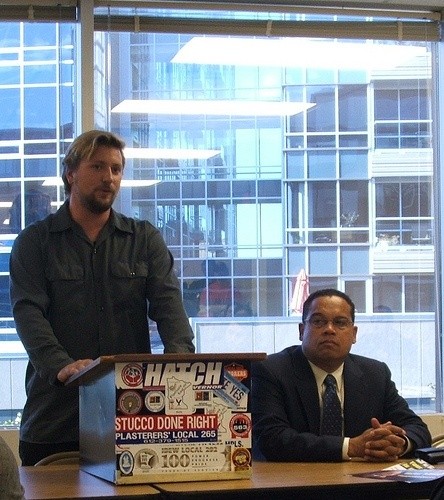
[303,313,353,330]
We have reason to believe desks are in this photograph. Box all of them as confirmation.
[19,459,444,500]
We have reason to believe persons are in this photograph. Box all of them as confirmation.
[8,130,196,468]
[252,289,432,463]
[189,261,251,320]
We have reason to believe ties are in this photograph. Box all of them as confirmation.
[322,374,342,436]
